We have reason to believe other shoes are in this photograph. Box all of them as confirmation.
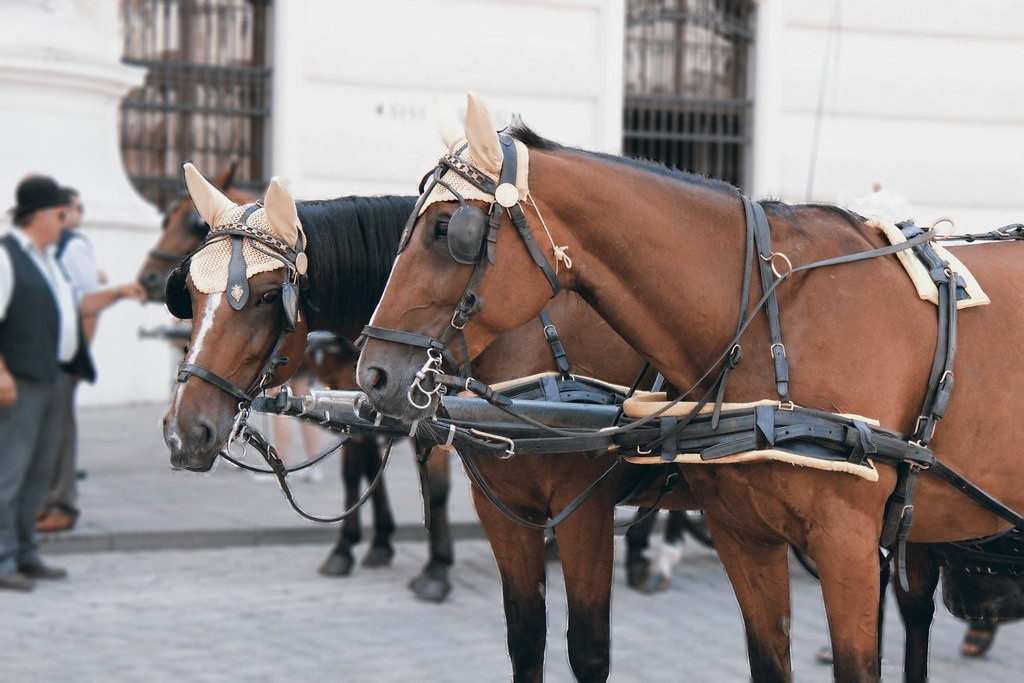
[0,572,33,588]
[22,561,65,577]
[35,512,71,530]
[296,464,322,481]
[252,473,286,482]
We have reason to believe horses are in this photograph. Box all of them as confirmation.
[137,91,1024,683]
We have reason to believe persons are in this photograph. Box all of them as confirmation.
[0,173,147,593]
[251,363,323,482]
[816,564,996,664]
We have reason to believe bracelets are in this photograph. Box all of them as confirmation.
[113,286,122,302]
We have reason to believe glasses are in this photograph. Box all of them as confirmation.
[48,212,65,221]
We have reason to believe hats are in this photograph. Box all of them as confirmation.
[9,176,71,214]
